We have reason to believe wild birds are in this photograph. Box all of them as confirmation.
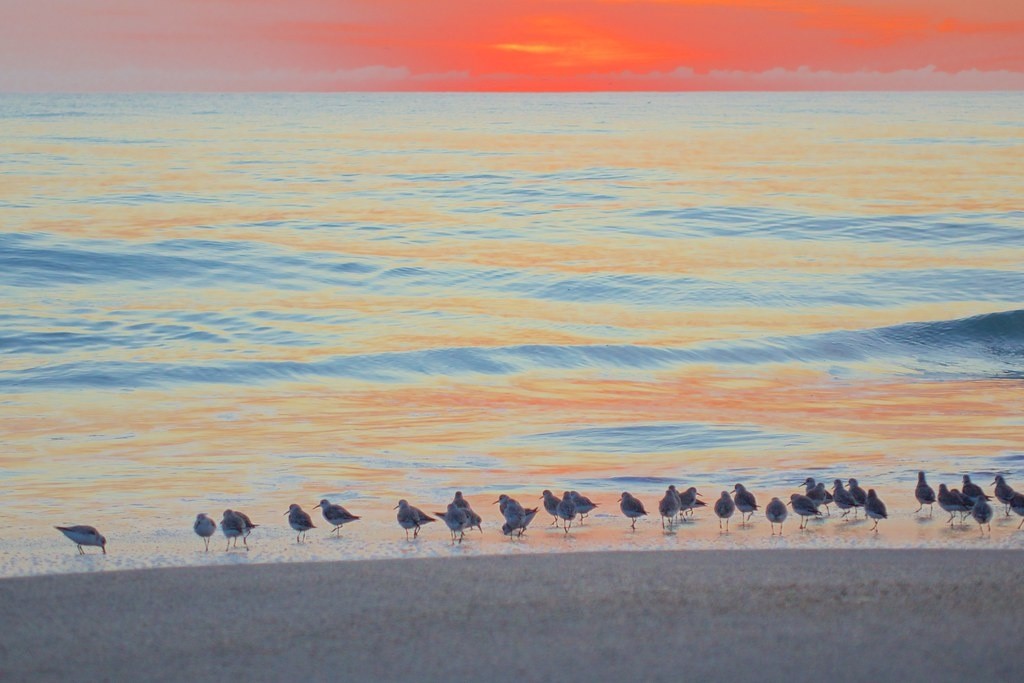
[659,485,708,527]
[492,495,539,536]
[540,489,600,528]
[220,510,260,551]
[194,513,216,551]
[54,525,107,555]
[787,477,888,532]
[393,491,482,540]
[618,492,649,527]
[914,471,1024,531]
[766,498,787,535]
[714,484,758,530]
[313,499,361,535]
[284,504,317,543]
